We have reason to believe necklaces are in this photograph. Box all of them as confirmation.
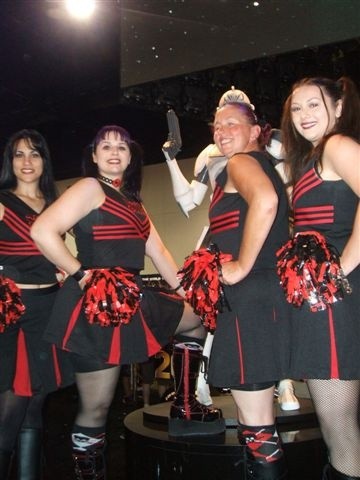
[98,174,120,187]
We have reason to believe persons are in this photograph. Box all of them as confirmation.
[202,102,293,480]
[281,77,360,480]
[0,129,75,480]
[162,89,300,411]
[28,125,223,480]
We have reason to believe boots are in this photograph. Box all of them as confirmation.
[168,334,225,436]
[70,424,107,480]
[244,453,289,480]
[10,429,44,480]
[0,449,15,480]
[321,461,360,480]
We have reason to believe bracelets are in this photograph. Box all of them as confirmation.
[72,265,87,281]
[175,285,181,291]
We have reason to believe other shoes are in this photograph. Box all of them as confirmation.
[278,377,300,411]
[196,372,213,406]
